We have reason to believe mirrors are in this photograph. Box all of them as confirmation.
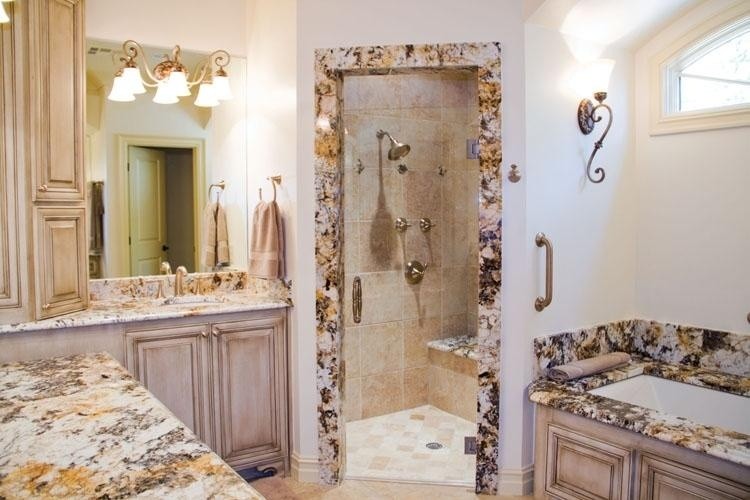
[82,36,251,276]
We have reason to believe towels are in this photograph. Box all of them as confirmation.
[204,202,230,270]
[547,350,634,383]
[246,201,285,282]
[91,179,105,251]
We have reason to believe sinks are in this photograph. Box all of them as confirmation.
[141,296,224,305]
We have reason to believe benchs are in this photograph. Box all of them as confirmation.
[527,357,750,500]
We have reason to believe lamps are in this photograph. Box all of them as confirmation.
[118,39,235,101]
[106,52,220,108]
[575,55,614,185]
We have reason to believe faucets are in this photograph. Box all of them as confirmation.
[174,266,187,296]
[160,262,172,275]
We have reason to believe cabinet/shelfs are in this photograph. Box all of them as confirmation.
[125,307,288,476]
